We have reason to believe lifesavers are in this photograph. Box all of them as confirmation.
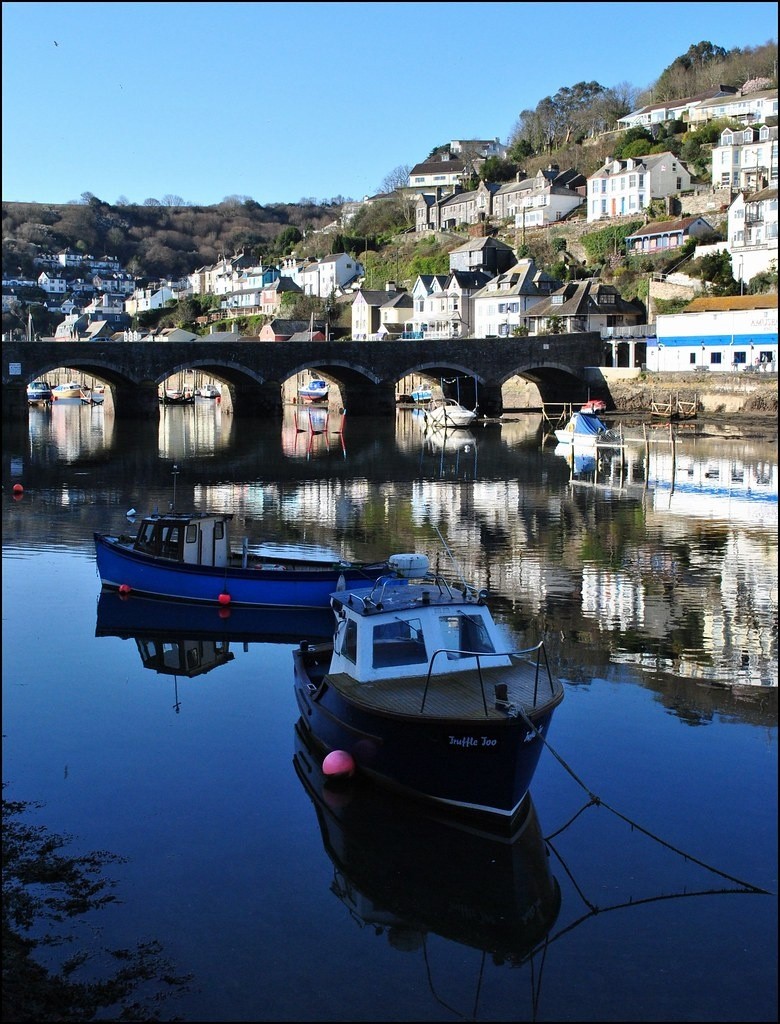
[605,430,615,442]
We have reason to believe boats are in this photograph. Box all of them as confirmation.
[423,423,475,450]
[395,384,432,405]
[94,586,340,714]
[554,441,600,474]
[298,380,329,401]
[293,523,565,818]
[94,458,430,612]
[292,589,561,1022]
[422,397,476,428]
[51,382,105,405]
[157,383,220,399]
[554,410,615,444]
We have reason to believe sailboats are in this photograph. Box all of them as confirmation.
[26,305,53,402]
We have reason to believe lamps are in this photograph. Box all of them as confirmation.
[476,589,489,605]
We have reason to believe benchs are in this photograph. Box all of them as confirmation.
[374,638,418,651]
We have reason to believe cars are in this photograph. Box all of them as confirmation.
[89,337,113,342]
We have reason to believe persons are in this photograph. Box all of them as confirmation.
[754,353,767,372]
[733,356,739,371]
